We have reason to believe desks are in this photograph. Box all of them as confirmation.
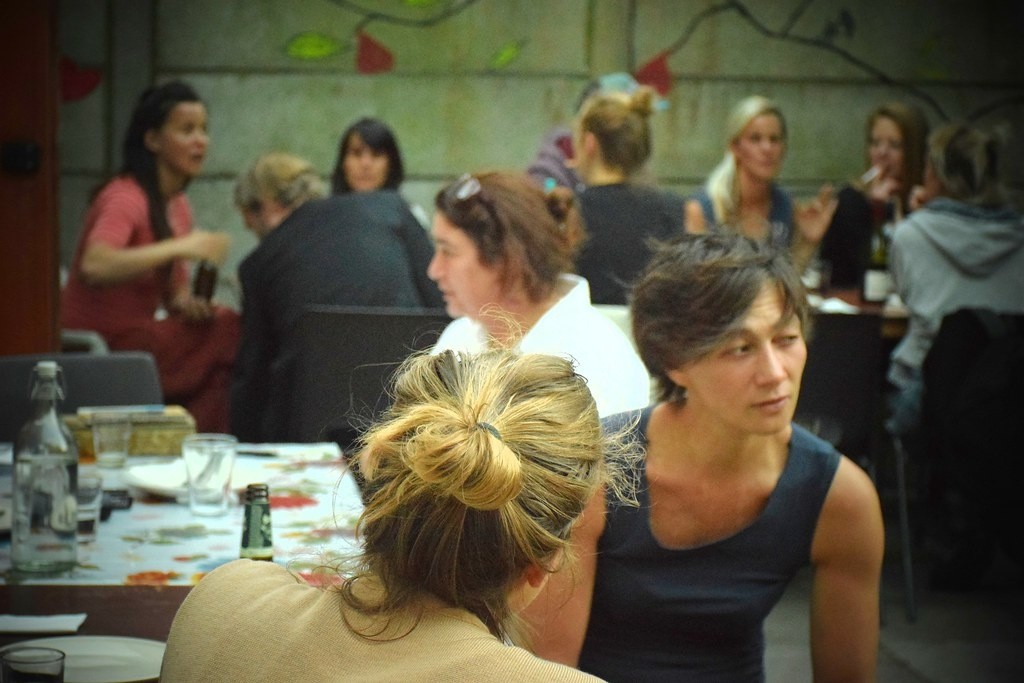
[0,442,374,683]
[805,285,910,323]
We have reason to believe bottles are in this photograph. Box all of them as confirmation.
[239,483,274,563]
[859,233,888,307]
[10,362,77,574]
[192,262,217,303]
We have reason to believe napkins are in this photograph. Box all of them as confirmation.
[0,613,88,632]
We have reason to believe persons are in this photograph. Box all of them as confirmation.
[331,117,403,196]
[428,169,651,416]
[832,105,931,280]
[889,118,1024,412]
[528,73,667,225]
[682,96,839,271]
[57,80,243,433]
[230,152,455,456]
[564,89,686,305]
[576,227,884,683]
[159,346,608,683]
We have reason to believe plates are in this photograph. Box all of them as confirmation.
[126,463,266,500]
[0,636,166,683]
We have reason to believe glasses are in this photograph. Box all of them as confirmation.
[448,174,502,231]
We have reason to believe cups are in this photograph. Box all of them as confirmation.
[91,412,131,469]
[0,647,65,683]
[181,434,236,515]
[76,471,103,545]
[803,260,824,293]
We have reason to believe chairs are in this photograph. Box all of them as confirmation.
[270,304,455,443]
[0,266,167,445]
[869,307,1024,622]
[596,301,666,406]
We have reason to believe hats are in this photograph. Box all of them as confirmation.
[577,71,669,114]
